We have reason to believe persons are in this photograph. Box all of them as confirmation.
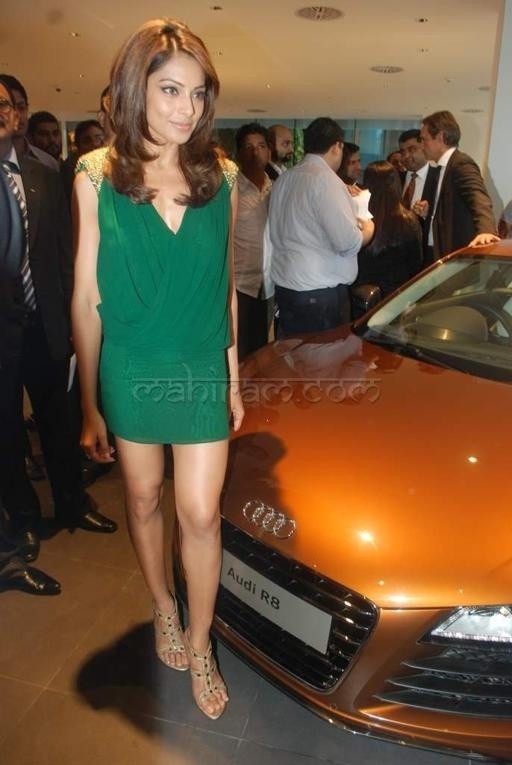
[0,79,118,563]
[388,151,407,172]
[336,141,367,190]
[395,130,438,262]
[234,123,275,364]
[25,109,64,430]
[62,120,109,163]
[269,117,374,341]
[264,125,293,183]
[1,74,71,480]
[71,19,244,720]
[100,84,117,146]
[350,160,424,322]
[0,161,63,594]
[414,111,502,271]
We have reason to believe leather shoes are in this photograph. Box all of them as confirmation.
[1,566,61,596]
[79,510,117,534]
[23,532,40,561]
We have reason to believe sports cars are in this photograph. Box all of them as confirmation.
[171,240,509,757]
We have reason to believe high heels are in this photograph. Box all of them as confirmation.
[152,591,189,671]
[183,626,229,719]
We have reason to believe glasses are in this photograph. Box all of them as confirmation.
[0,101,14,114]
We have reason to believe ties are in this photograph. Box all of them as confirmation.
[0,160,37,310]
[403,173,417,210]
